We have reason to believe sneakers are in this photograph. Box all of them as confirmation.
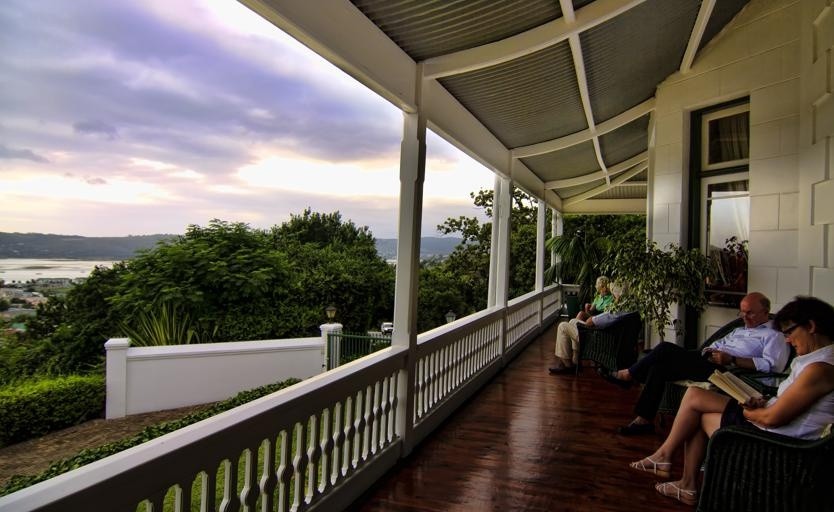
[549,362,577,376]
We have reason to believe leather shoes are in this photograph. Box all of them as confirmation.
[597,367,633,390]
[616,422,650,437]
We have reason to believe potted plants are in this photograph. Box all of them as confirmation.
[544,224,617,321]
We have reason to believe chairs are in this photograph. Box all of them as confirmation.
[656,312,833,512]
[576,312,640,376]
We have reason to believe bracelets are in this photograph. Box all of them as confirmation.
[731,355,736,369]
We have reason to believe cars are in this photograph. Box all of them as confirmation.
[381,322,393,335]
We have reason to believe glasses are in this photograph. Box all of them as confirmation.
[783,323,801,338]
[736,309,766,320]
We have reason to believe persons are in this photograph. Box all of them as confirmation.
[630,295,834,505]
[597,292,792,438]
[548,283,636,374]
[576,276,616,321]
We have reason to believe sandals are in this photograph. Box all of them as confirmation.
[628,457,673,479]
[654,481,698,505]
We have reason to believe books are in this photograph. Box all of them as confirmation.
[706,369,764,404]
